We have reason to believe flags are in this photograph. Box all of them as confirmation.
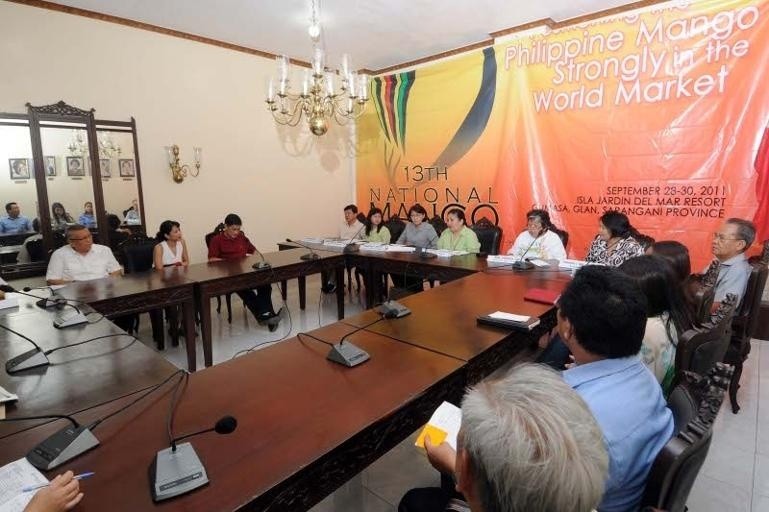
[754,117,769,244]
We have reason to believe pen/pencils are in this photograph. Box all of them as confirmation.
[20,471,94,494]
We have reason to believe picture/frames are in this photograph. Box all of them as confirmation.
[87,160,111,179]
[42,154,57,177]
[118,158,136,177]
[68,157,86,177]
[9,157,29,180]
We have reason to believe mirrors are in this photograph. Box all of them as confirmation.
[0,98,149,282]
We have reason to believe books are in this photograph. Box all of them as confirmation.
[476,309,540,331]
[302,233,605,273]
[524,286,563,305]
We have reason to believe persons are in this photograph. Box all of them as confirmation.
[121,161,132,175]
[322,203,367,294]
[100,162,110,177]
[585,211,645,268]
[206,213,287,332]
[44,226,136,336]
[360,209,391,294]
[153,219,204,341]
[13,159,27,177]
[505,209,567,260]
[436,211,481,255]
[124,200,141,224]
[450,361,611,512]
[394,205,440,290]
[17,214,67,263]
[647,239,704,336]
[397,266,674,511]
[106,212,133,245]
[0,201,30,234]
[51,203,78,230]
[45,158,54,175]
[22,470,85,511]
[701,218,756,315]
[74,201,97,228]
[538,255,678,390]
[69,158,82,176]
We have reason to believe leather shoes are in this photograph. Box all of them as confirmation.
[268,308,282,331]
[258,313,281,325]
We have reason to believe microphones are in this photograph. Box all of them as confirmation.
[326,309,399,367]
[286,238,321,261]
[343,221,369,253]
[419,227,448,259]
[0,413,100,471]
[0,325,49,374]
[0,284,88,328]
[512,225,551,270]
[147,415,236,503]
[23,287,67,307]
[382,273,437,318]
[244,237,272,269]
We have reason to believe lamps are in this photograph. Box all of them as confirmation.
[164,139,202,183]
[264,1,372,142]
[66,126,121,159]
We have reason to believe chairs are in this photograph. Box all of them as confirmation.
[346,212,502,297]
[629,238,769,512]
[113,232,182,346]
[465,216,504,257]
[546,222,570,253]
[203,220,248,323]
